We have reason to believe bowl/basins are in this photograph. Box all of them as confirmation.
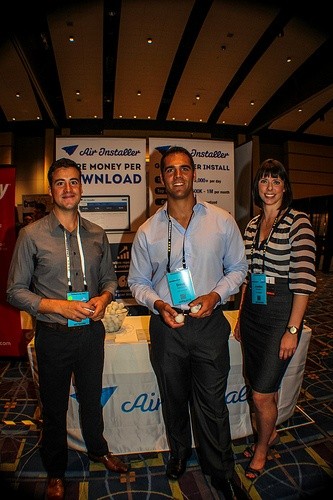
[100,306,129,333]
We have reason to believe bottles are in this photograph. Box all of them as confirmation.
[112,250,130,271]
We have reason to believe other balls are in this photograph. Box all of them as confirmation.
[101,301,128,333]
[190,305,199,313]
[175,313,185,324]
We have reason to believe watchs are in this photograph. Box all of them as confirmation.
[287,326,299,335]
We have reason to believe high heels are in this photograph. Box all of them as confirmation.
[243,434,279,458]
[246,463,265,480]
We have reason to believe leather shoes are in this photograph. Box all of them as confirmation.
[45,479,63,500]
[88,452,128,473]
[165,455,187,479]
[210,473,251,500]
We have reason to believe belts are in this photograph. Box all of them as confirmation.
[170,305,222,315]
[36,318,100,331]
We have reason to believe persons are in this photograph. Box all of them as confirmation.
[7,158,130,499]
[232,158,317,478]
[128,147,249,500]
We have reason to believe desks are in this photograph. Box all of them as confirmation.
[27,310,312,456]
